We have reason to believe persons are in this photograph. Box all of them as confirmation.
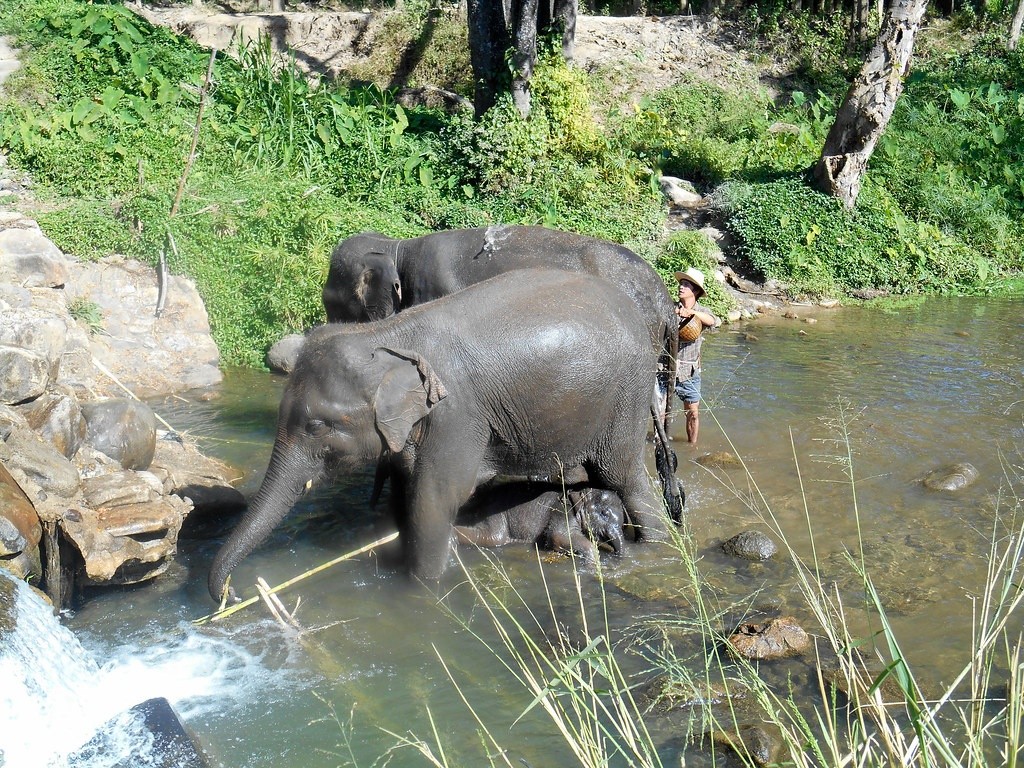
[653,267,722,443]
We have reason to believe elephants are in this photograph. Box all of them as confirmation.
[208,229,681,603]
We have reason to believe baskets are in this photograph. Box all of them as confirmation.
[677,314,702,341]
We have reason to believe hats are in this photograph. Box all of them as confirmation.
[676,266,707,298]
